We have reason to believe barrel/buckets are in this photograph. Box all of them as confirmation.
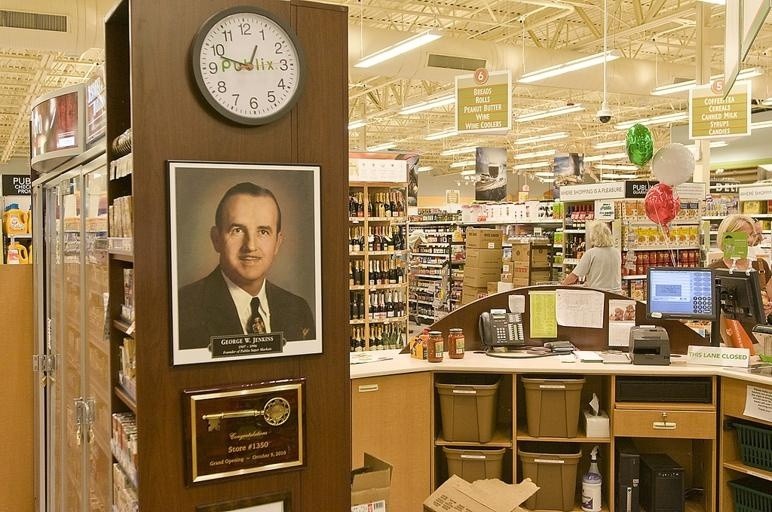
[3,203,32,264]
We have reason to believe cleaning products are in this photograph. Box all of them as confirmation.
[581,444,604,512]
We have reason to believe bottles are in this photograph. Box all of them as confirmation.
[565,203,594,284]
[518,224,564,243]
[548,248,563,281]
[580,472,602,512]
[462,200,564,220]
[346,189,406,354]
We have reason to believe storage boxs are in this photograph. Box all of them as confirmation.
[436,439,508,482]
[349,451,395,512]
[436,380,501,444]
[460,223,553,308]
[422,472,543,512]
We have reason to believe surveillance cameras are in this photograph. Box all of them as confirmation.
[596,102,614,123]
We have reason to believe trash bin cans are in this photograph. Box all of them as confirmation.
[442,446,507,482]
[434,374,504,443]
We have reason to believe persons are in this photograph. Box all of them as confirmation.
[179,181,317,350]
[562,220,623,294]
[707,212,772,323]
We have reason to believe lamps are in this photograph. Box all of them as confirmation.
[649,47,764,97]
[516,4,621,84]
[514,90,587,123]
[346,123,771,180]
[400,78,457,116]
[613,97,689,131]
[762,51,772,106]
[353,4,442,70]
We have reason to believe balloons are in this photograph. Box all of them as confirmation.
[651,144,694,189]
[645,182,681,226]
[625,123,654,167]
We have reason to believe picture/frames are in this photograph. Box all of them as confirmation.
[163,156,329,370]
[182,375,309,488]
[197,491,296,512]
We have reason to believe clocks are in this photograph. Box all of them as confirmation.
[191,5,307,130]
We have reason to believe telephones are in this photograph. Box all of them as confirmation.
[478,308,525,346]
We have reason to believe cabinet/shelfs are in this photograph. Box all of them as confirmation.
[552,190,709,309]
[346,174,413,357]
[432,346,616,511]
[344,342,435,511]
[696,204,734,255]
[405,218,466,328]
[100,1,357,511]
[609,349,719,510]
[713,351,771,512]
[28,141,115,511]
[734,182,772,280]
[467,220,563,260]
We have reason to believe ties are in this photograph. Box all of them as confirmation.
[247,298,266,333]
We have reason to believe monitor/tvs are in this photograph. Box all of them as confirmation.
[715,268,764,344]
[646,267,717,321]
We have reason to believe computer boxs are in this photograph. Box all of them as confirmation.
[617,445,640,512]
[638,454,685,512]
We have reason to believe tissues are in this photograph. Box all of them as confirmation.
[582,391,609,437]
